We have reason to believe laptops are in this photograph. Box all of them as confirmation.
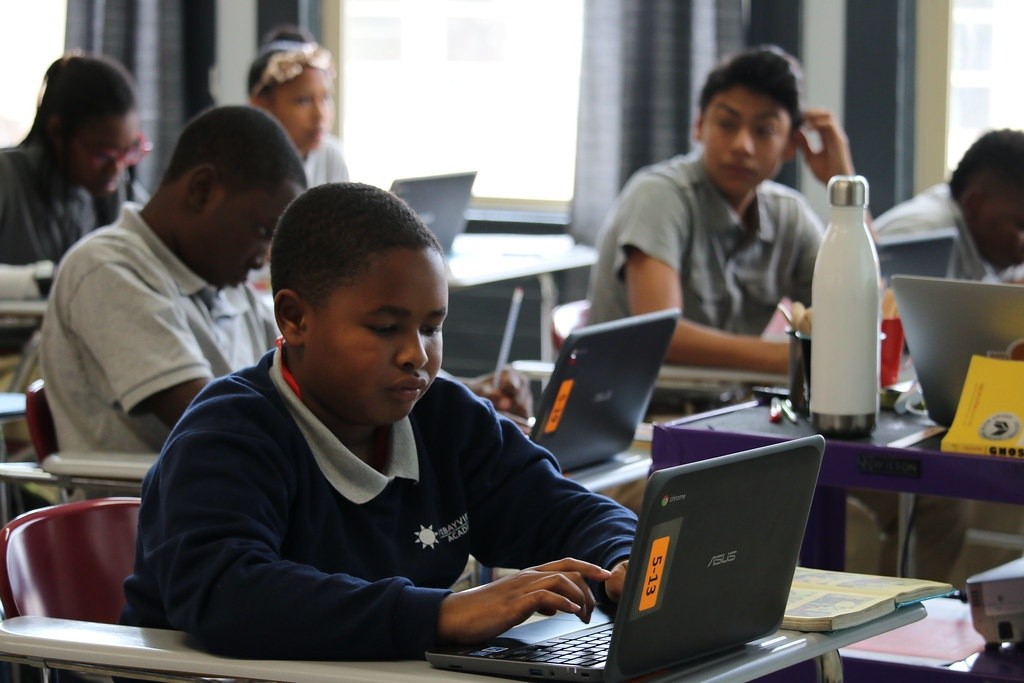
[388,172,476,255]
[878,231,1024,427]
[425,435,827,683]
[529,308,686,472]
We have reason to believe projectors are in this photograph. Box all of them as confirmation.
[965,556,1024,653]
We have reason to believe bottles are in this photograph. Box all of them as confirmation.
[808,176,882,435]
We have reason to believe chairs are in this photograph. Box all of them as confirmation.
[0,495,147,620]
[25,377,61,467]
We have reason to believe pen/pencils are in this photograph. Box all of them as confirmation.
[782,399,797,423]
[492,288,524,388]
[770,397,781,423]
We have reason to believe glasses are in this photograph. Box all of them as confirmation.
[252,42,331,100]
[72,130,150,171]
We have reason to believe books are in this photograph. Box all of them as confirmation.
[941,354,1024,460]
[779,566,957,632]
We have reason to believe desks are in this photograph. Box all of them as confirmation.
[652,390,1024,571]
[0,605,927,683]
[438,230,602,393]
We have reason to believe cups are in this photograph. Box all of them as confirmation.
[788,328,809,417]
[512,358,555,419]
[881,319,901,388]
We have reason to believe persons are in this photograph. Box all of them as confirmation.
[869,127,1024,356]
[40,105,308,501]
[117,182,640,664]
[0,50,150,299]
[245,28,350,263]
[586,44,853,417]
